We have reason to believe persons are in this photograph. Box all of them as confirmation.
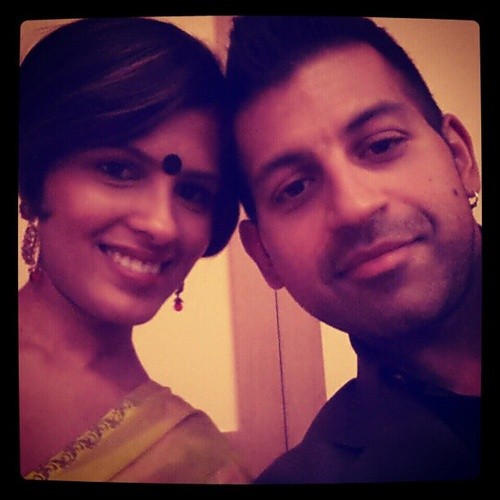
[20,16,253,485]
[224,16,482,485]
